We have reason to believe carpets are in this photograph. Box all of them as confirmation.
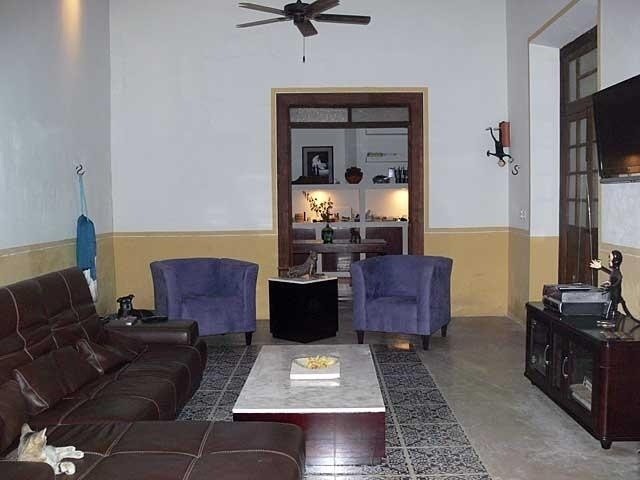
[174,344,494,480]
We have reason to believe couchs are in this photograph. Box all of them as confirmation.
[0,267,306,480]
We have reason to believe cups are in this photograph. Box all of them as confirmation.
[295,212,304,223]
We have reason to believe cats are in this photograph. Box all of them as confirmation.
[5,422,84,476]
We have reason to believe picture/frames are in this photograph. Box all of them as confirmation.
[301,145,335,184]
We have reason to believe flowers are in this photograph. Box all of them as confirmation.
[301,190,334,223]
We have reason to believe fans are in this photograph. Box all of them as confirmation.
[235,0,372,38]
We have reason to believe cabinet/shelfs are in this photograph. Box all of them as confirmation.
[523,300,640,450]
[291,181,410,298]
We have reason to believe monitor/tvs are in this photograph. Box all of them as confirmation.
[592,75,640,184]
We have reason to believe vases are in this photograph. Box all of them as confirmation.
[321,223,334,244]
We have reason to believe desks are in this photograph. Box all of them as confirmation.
[267,275,340,344]
[292,239,388,254]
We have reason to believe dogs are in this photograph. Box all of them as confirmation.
[117,294,155,319]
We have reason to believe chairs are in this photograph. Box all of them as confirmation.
[349,254,453,350]
[150,256,259,346]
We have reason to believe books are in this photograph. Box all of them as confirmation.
[289,354,342,380]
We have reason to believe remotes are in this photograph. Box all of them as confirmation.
[142,315,167,322]
[126,316,137,326]
[558,286,590,292]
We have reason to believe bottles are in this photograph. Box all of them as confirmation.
[322,224,334,240]
[326,210,408,222]
[325,231,332,241]
[389,165,407,183]
